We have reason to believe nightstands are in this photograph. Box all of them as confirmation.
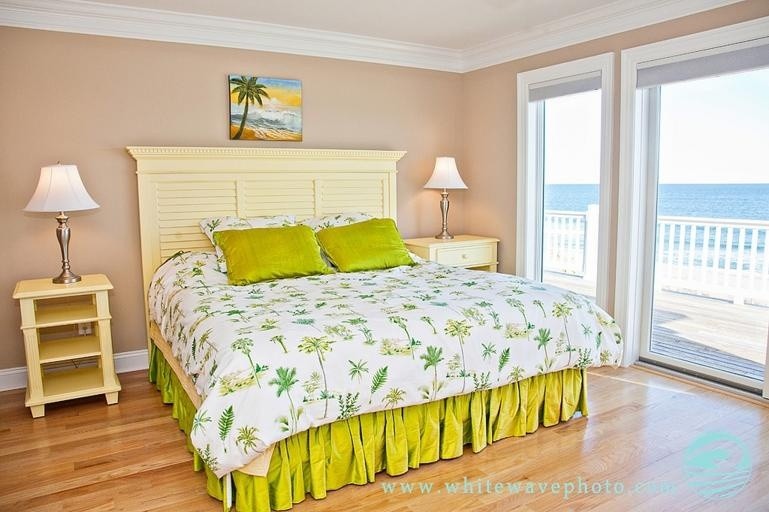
[402,234,500,275]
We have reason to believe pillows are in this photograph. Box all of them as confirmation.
[198,212,422,290]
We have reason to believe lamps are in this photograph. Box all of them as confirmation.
[22,162,100,285]
[423,156,470,240]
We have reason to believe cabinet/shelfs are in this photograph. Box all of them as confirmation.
[12,273,123,420]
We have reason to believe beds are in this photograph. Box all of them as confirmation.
[126,142,592,512]
